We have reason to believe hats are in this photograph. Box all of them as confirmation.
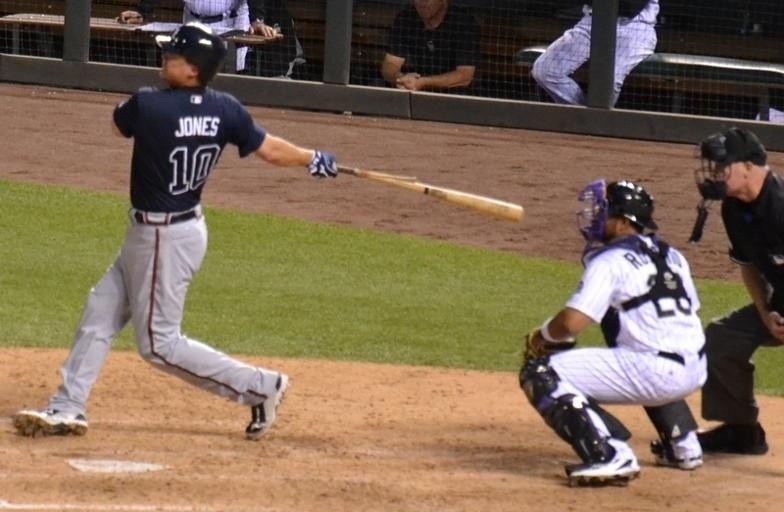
[155,21,227,68]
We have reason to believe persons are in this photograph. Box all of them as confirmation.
[115,0,278,77]
[13,21,340,443]
[378,1,480,91]
[516,176,713,488]
[695,126,783,455]
[530,1,661,109]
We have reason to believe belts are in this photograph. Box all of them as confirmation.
[191,10,237,23]
[133,211,196,227]
[661,346,707,364]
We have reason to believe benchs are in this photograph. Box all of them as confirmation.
[515,43,784,122]
[1,13,283,73]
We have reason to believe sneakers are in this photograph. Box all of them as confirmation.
[13,406,88,436]
[696,422,771,457]
[245,370,290,439]
[567,437,642,487]
[649,431,703,471]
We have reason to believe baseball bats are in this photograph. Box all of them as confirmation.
[336,166,525,224]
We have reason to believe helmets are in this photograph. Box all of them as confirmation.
[694,125,767,198]
[580,177,658,244]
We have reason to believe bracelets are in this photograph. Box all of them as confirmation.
[539,317,575,345]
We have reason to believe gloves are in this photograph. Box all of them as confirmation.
[307,149,339,180]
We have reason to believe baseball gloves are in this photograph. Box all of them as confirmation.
[523,325,577,363]
